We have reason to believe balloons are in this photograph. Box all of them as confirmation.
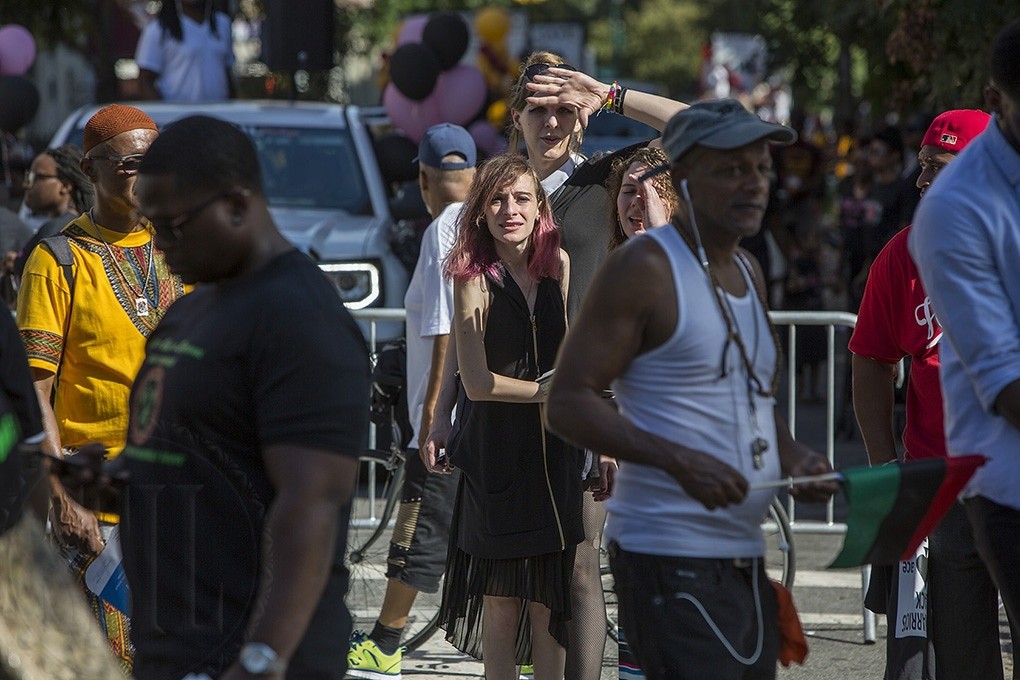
[382,11,519,151]
[1,77,40,134]
[0,24,35,77]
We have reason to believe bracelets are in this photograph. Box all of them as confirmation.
[606,80,628,117]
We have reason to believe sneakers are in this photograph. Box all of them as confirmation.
[344,630,402,680]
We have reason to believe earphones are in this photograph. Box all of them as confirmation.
[679,177,694,202]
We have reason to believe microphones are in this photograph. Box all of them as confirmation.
[699,246,708,265]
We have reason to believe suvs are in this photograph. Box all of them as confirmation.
[29,99,409,416]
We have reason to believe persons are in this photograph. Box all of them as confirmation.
[14,104,197,679]
[344,121,533,679]
[607,148,677,255]
[422,51,692,680]
[440,152,583,680]
[544,98,840,680]
[908,22,1020,680]
[135,0,238,102]
[770,107,936,405]
[3,142,96,291]
[848,107,1020,680]
[1,293,45,454]
[113,116,374,680]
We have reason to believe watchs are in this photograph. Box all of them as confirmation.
[237,641,290,678]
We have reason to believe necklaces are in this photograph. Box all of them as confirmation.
[690,240,768,468]
[671,217,783,396]
[89,204,154,317]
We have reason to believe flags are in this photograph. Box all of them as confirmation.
[825,454,986,570]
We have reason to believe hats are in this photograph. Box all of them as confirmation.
[84,102,157,155]
[412,121,478,170]
[920,105,995,150]
[661,98,795,164]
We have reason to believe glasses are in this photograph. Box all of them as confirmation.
[518,63,578,91]
[85,153,144,176]
[149,188,238,238]
[24,172,61,188]
